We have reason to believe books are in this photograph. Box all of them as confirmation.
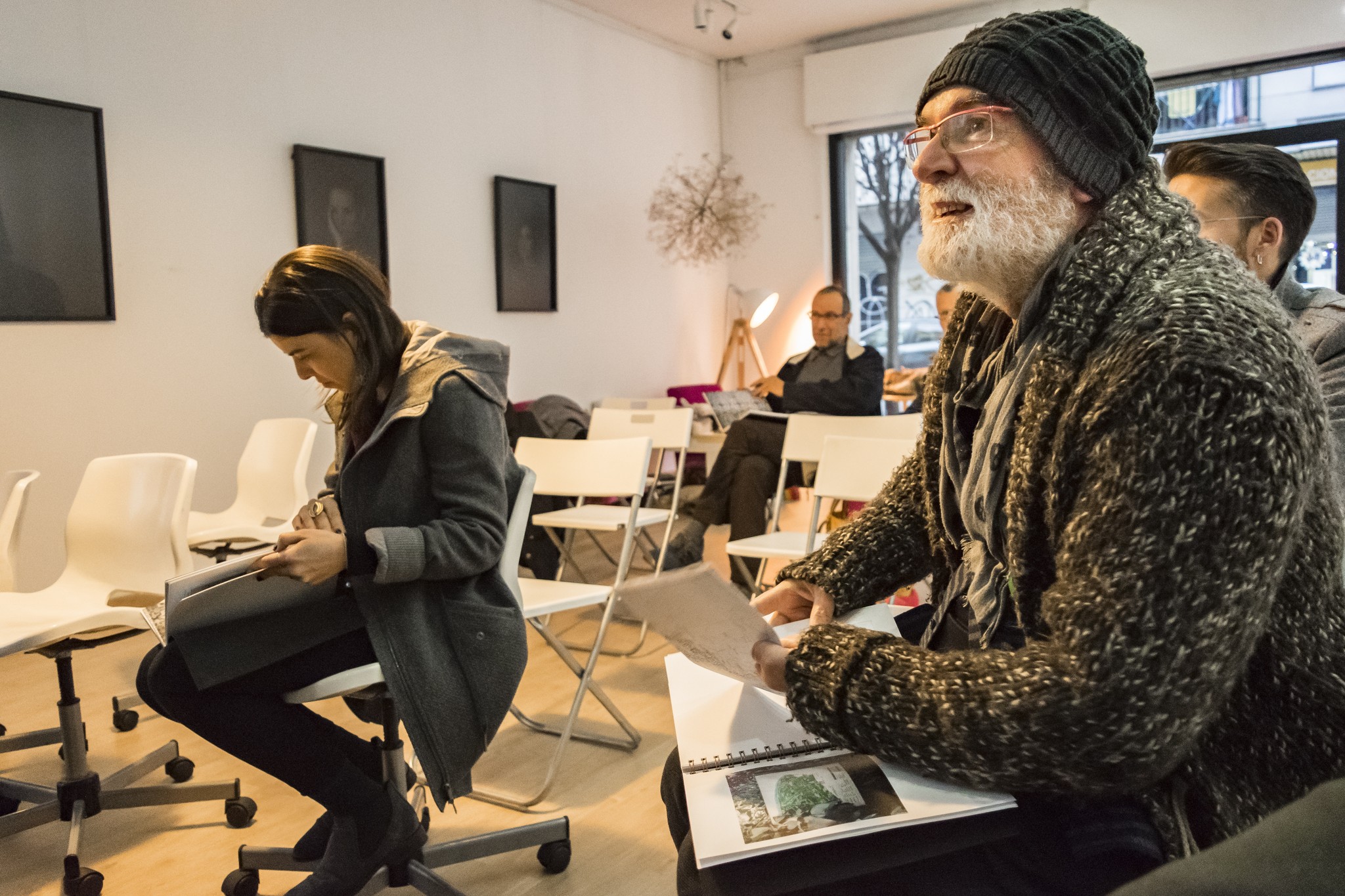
[666,602,1018,869]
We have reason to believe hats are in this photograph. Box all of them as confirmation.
[915,6,1159,205]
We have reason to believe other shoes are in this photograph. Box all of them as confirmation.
[286,781,428,896]
[291,735,416,862]
[650,534,702,568]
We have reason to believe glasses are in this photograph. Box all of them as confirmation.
[808,311,846,320]
[905,108,1017,171]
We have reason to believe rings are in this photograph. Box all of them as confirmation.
[309,502,324,517]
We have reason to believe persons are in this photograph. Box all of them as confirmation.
[660,5,1345,896]
[1161,141,1345,447]
[136,246,527,896]
[647,286,884,599]
[902,283,963,415]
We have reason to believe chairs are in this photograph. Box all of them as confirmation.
[0,386,924,896]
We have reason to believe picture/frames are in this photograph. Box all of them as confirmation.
[0,90,115,321]
[494,176,558,313]
[294,143,389,284]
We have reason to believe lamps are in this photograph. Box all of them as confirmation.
[717,284,779,391]
[694,0,739,40]
